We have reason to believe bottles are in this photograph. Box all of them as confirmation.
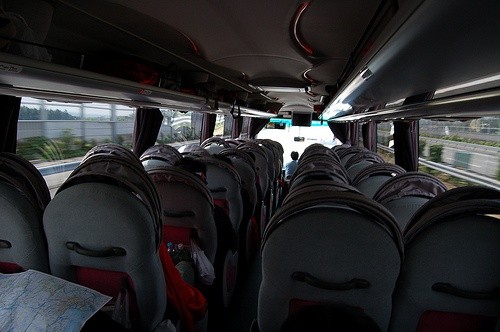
[178,244,190,261]
[167,242,179,265]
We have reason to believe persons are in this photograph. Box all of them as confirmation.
[284,151,299,180]
[139,144,264,332]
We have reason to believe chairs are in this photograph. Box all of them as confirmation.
[0,137,500,332]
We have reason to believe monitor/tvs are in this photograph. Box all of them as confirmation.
[292,111,312,127]
